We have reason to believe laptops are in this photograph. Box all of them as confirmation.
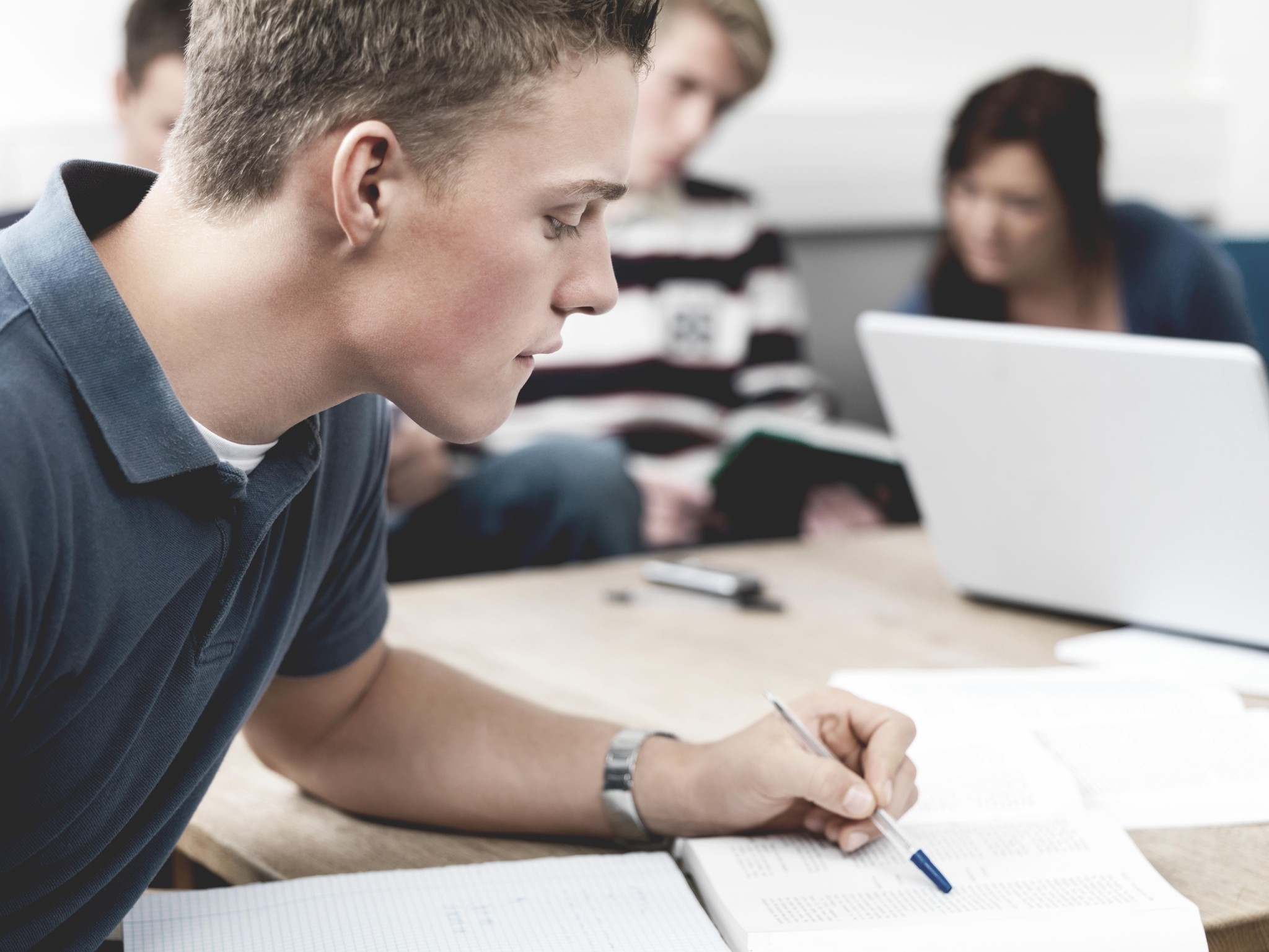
[850,308,1269,653]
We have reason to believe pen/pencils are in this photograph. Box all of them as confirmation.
[773,699,953,893]
[642,559,781,610]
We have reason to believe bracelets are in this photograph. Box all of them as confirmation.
[603,724,676,835]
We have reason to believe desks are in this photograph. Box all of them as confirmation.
[177,524,1269,952]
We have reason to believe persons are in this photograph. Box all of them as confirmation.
[0,0,892,585]
[902,67,1260,353]
[0,0,918,952]
